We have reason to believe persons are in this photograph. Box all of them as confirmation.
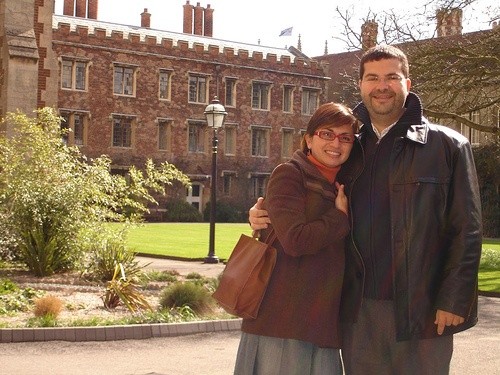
[234,101,360,375]
[248,43,483,375]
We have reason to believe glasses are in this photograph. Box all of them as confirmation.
[313,131,355,143]
[361,75,406,84]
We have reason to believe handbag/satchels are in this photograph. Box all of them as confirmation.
[211,159,306,320]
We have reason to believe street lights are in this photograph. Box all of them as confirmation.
[203,95,228,263]
